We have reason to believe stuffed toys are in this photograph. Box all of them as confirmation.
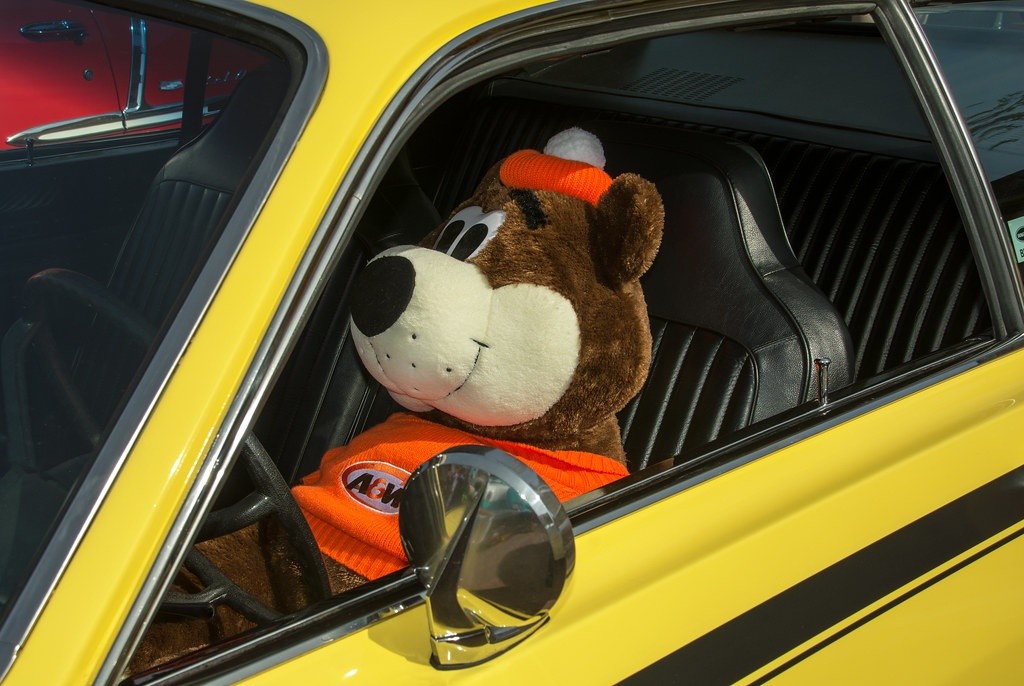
[124,126,661,675]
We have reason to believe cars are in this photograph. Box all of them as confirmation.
[0,0,1023,686]
[0,0,270,150]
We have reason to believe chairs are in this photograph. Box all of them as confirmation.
[83,51,855,518]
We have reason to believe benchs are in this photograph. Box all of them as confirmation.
[435,97,988,386]
